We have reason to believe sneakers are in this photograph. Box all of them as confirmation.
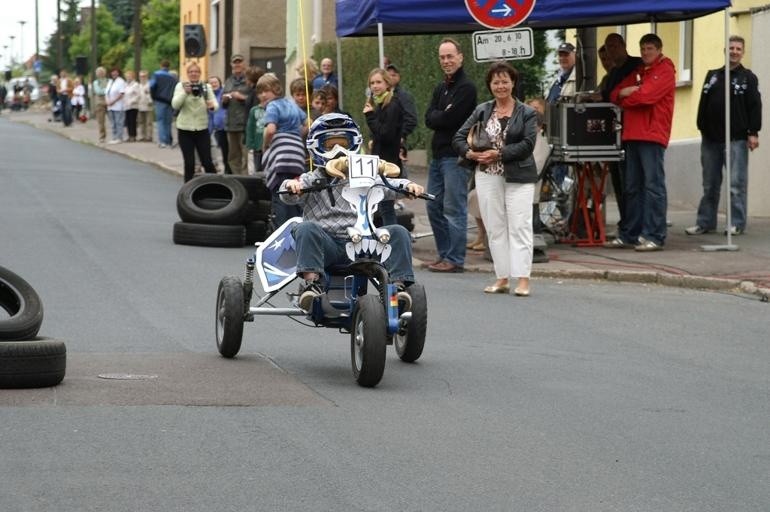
[683,220,717,237]
[388,282,414,315]
[601,215,676,252]
[723,221,746,238]
[299,282,323,315]
[465,241,488,253]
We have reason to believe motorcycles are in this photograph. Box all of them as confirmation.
[215,172,440,387]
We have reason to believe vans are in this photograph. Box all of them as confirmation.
[3,74,40,106]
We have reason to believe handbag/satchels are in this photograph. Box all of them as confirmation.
[456,117,495,171]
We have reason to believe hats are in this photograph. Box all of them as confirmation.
[386,62,401,76]
[557,42,578,55]
[229,52,245,65]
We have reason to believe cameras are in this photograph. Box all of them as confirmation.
[189,82,204,97]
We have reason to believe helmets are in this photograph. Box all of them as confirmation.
[304,111,366,171]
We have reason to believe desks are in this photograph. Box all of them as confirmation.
[548,152,626,246]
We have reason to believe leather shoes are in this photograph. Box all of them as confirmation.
[514,281,532,297]
[483,279,512,294]
[421,256,466,275]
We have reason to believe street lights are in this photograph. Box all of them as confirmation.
[0,19,28,69]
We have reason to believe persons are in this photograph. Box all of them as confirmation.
[246,54,351,233]
[2,79,32,112]
[363,54,417,177]
[546,34,675,252]
[466,98,547,250]
[279,114,424,318]
[685,37,761,235]
[92,58,178,147]
[425,38,476,273]
[49,72,86,127]
[453,62,537,294]
[171,54,246,183]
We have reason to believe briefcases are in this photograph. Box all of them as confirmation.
[546,91,624,152]
